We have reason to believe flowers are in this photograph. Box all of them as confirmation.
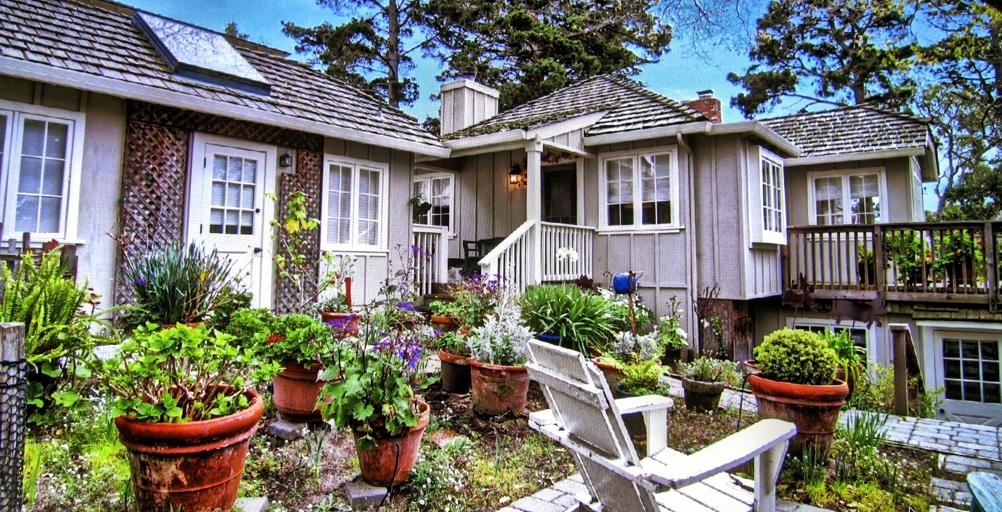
[655,297,688,348]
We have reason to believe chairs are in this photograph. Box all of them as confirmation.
[526,333,797,512]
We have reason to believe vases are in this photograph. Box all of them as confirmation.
[661,347,681,368]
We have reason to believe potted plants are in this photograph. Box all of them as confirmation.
[3,229,292,512]
[676,357,731,415]
[745,326,872,468]
[310,243,443,485]
[857,231,976,283]
[426,268,673,443]
[260,188,343,423]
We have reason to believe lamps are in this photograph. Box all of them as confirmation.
[508,163,523,185]
[280,153,292,167]
